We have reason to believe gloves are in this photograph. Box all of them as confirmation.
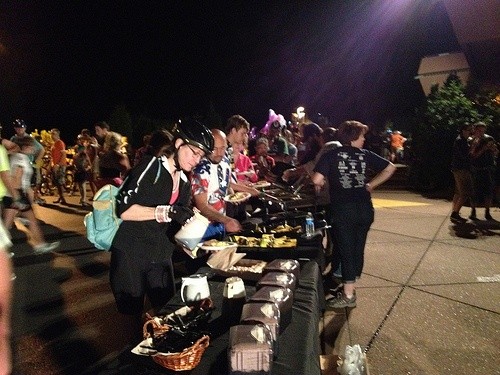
[155,204,195,225]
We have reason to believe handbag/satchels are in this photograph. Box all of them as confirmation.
[82,156,156,252]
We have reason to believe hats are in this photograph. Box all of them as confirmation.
[256,138,268,147]
[302,123,320,143]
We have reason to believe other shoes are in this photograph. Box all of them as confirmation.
[450,212,466,225]
[469,213,476,220]
[328,272,343,279]
[88,196,94,201]
[34,241,60,256]
[79,198,86,204]
[33,184,74,206]
[485,213,493,220]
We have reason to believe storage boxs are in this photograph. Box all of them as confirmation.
[255,271,297,300]
[262,258,301,289]
[226,326,274,375]
[246,286,294,328]
[239,303,280,357]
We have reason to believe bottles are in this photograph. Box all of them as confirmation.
[306,212,314,236]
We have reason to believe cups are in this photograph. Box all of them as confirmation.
[174,212,209,251]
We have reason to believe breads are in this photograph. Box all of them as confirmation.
[233,225,301,248]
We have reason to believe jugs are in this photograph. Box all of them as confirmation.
[181,274,210,306]
[222,276,247,318]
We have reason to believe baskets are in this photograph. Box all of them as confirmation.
[142,320,210,372]
[145,298,214,337]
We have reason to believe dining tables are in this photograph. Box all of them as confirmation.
[80,185,332,375]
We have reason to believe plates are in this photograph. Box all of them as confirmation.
[198,242,237,250]
[249,182,271,187]
[224,192,251,203]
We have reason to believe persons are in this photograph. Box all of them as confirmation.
[449,123,500,223]
[0,115,397,375]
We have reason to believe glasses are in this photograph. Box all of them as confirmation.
[187,145,205,160]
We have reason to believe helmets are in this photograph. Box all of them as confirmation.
[173,119,215,155]
[13,119,28,128]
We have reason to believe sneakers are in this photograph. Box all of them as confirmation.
[327,286,357,309]
